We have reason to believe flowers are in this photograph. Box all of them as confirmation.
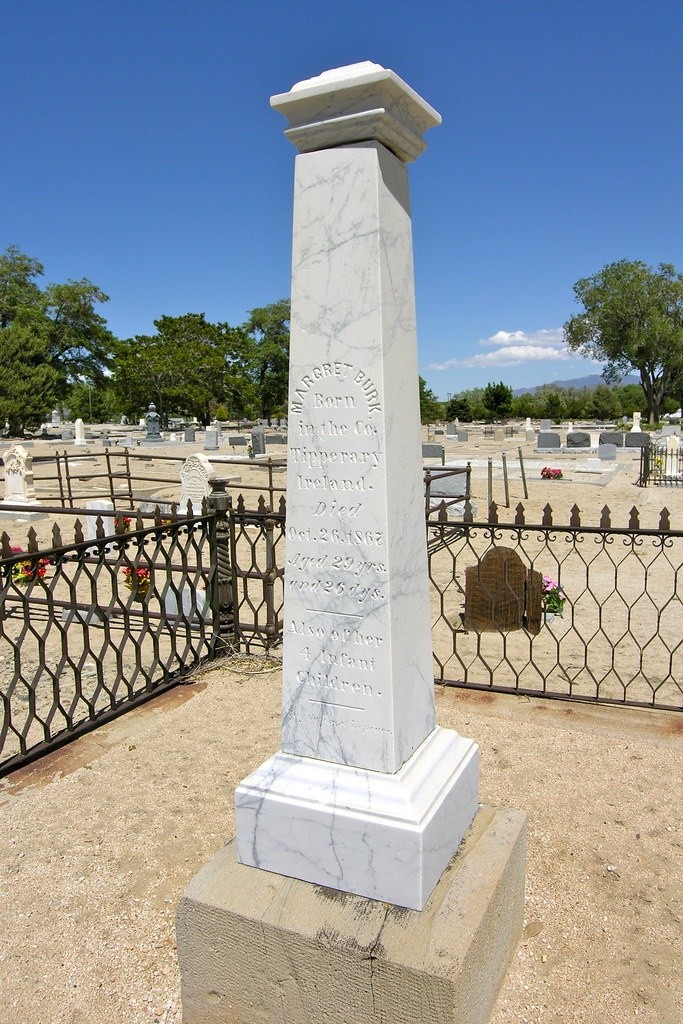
[541,576,566,619]
[10,546,51,585]
[541,467,563,479]
[122,565,150,594]
[114,515,132,543]
